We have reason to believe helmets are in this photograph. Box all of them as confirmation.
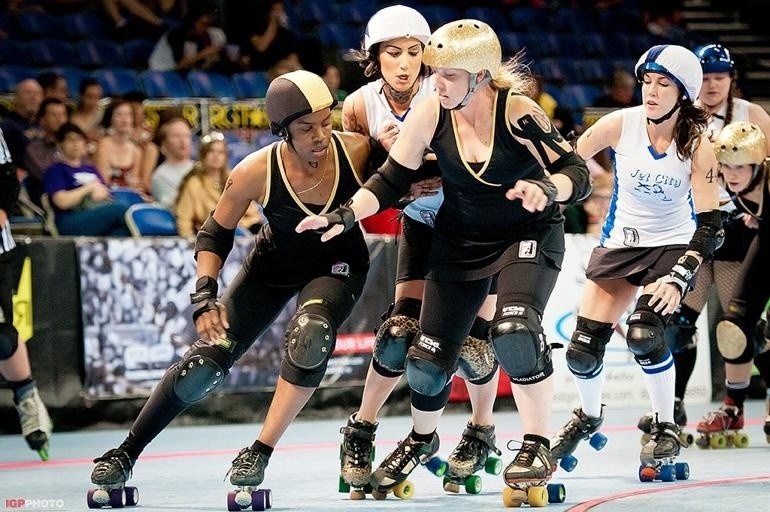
[422,17,503,81]
[694,41,736,73]
[363,3,432,62]
[713,120,768,166]
[263,68,336,136]
[633,43,705,104]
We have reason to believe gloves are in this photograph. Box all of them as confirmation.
[524,178,558,208]
[189,274,221,324]
[364,137,389,172]
[312,199,357,236]
[663,254,701,299]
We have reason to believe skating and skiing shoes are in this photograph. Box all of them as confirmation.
[369,431,449,499]
[637,400,695,446]
[227,445,274,511]
[638,422,690,482]
[502,439,566,508]
[11,378,56,461]
[551,403,608,473]
[85,447,140,509]
[695,395,750,450]
[443,416,503,494]
[338,410,380,500]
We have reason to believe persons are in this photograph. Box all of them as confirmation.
[1,1,339,91]
[550,44,725,482]
[43,122,134,236]
[295,19,595,506]
[88,70,442,508]
[695,121,770,448]
[174,128,266,249]
[0,128,53,462]
[94,96,154,203]
[591,68,641,110]
[71,79,117,157]
[141,140,166,195]
[339,4,500,499]
[4,79,44,171]
[500,1,686,35]
[36,71,77,120]
[148,116,198,207]
[637,44,770,448]
[26,96,69,172]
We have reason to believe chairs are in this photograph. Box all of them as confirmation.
[109,189,146,239]
[0,66,39,93]
[0,9,188,68]
[232,71,269,98]
[497,9,665,123]
[10,194,46,234]
[189,132,284,170]
[288,1,378,70]
[90,68,141,97]
[465,7,506,30]
[129,203,179,238]
[187,70,238,96]
[54,66,89,100]
[419,4,461,32]
[142,69,191,99]
